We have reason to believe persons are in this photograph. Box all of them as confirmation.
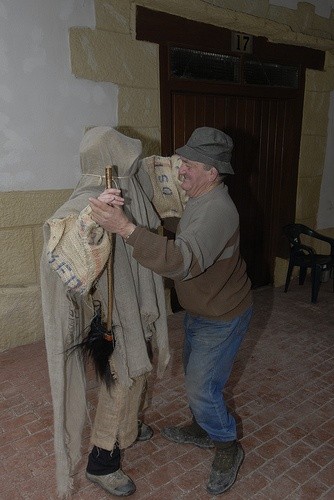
[88,126,256,496]
[40,125,192,498]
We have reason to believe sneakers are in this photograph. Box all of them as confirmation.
[206,446,244,495]
[85,468,136,497]
[135,422,153,440]
[162,425,215,449]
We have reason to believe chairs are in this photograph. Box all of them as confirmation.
[281,223,334,303]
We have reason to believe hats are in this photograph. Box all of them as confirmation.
[177,127,234,175]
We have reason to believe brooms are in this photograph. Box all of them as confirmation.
[62,165,117,398]
